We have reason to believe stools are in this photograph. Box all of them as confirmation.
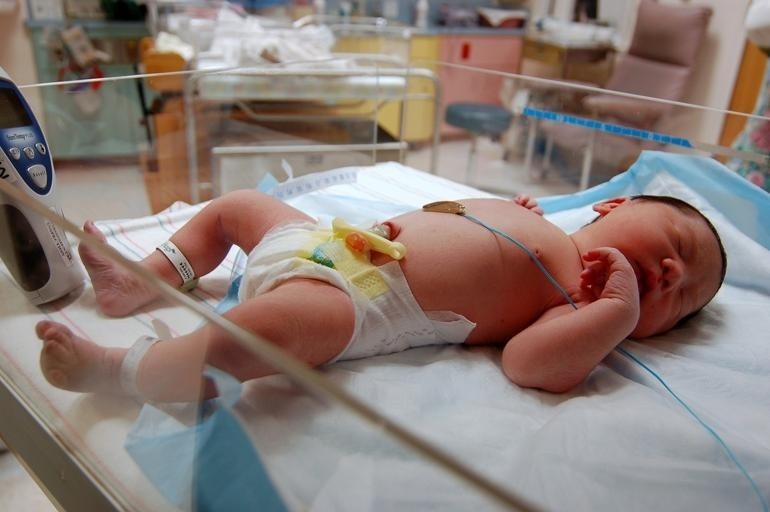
[442,102,518,198]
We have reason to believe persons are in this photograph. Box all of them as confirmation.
[34,180,728,406]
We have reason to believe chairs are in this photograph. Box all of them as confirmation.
[523,1,715,191]
